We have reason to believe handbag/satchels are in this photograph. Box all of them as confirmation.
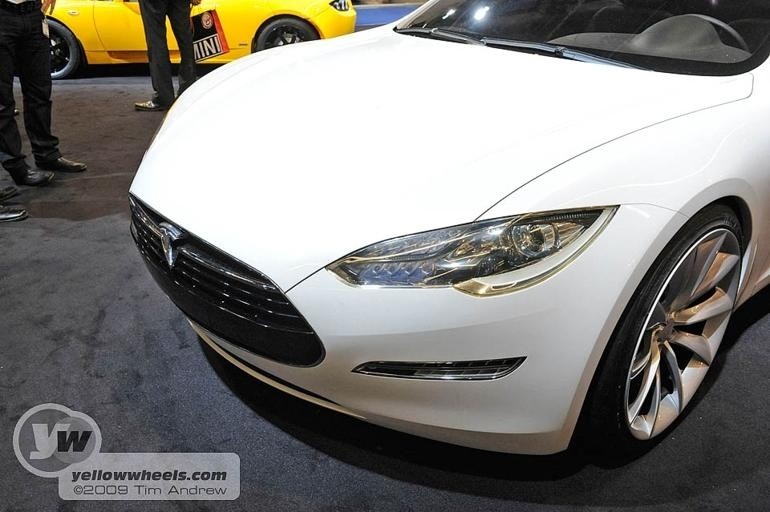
[190,4,230,62]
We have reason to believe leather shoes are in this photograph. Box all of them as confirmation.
[15,167,52,186]
[36,157,86,172]
[1,186,18,201]
[0,207,28,223]
[134,100,167,111]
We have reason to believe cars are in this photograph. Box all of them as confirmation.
[130,0,769,459]
[41,0,357,89]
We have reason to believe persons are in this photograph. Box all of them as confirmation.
[134,0,202,112]
[0,186,30,222]
[1,0,86,188]
